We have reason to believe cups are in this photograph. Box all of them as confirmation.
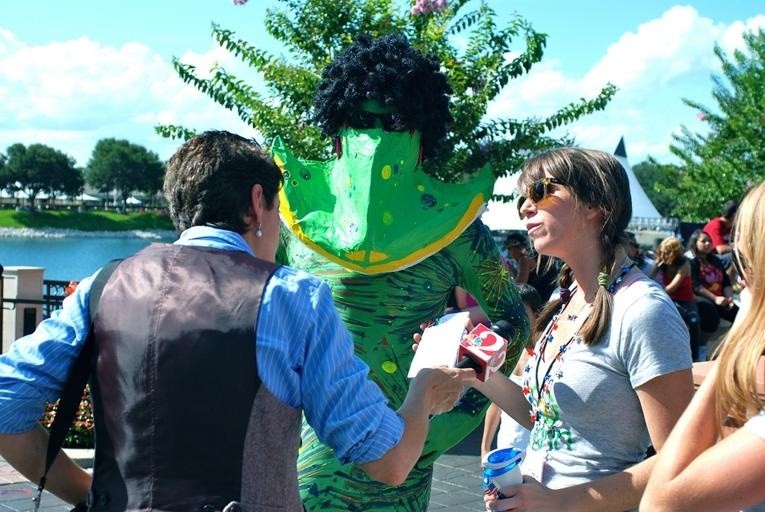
[483,444,528,500]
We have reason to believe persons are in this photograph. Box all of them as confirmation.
[617,230,664,284]
[444,225,572,347]
[701,199,748,294]
[685,229,741,333]
[412,146,697,511]
[0,130,479,511]
[636,178,765,512]
[647,237,709,363]
[480,282,544,471]
[248,30,529,510]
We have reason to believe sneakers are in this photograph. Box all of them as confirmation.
[698,345,709,362]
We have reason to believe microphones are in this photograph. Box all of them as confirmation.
[456,318,516,368]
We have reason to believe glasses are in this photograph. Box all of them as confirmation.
[517,177,568,221]
[730,247,748,281]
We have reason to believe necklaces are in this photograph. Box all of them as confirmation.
[566,283,598,322]
